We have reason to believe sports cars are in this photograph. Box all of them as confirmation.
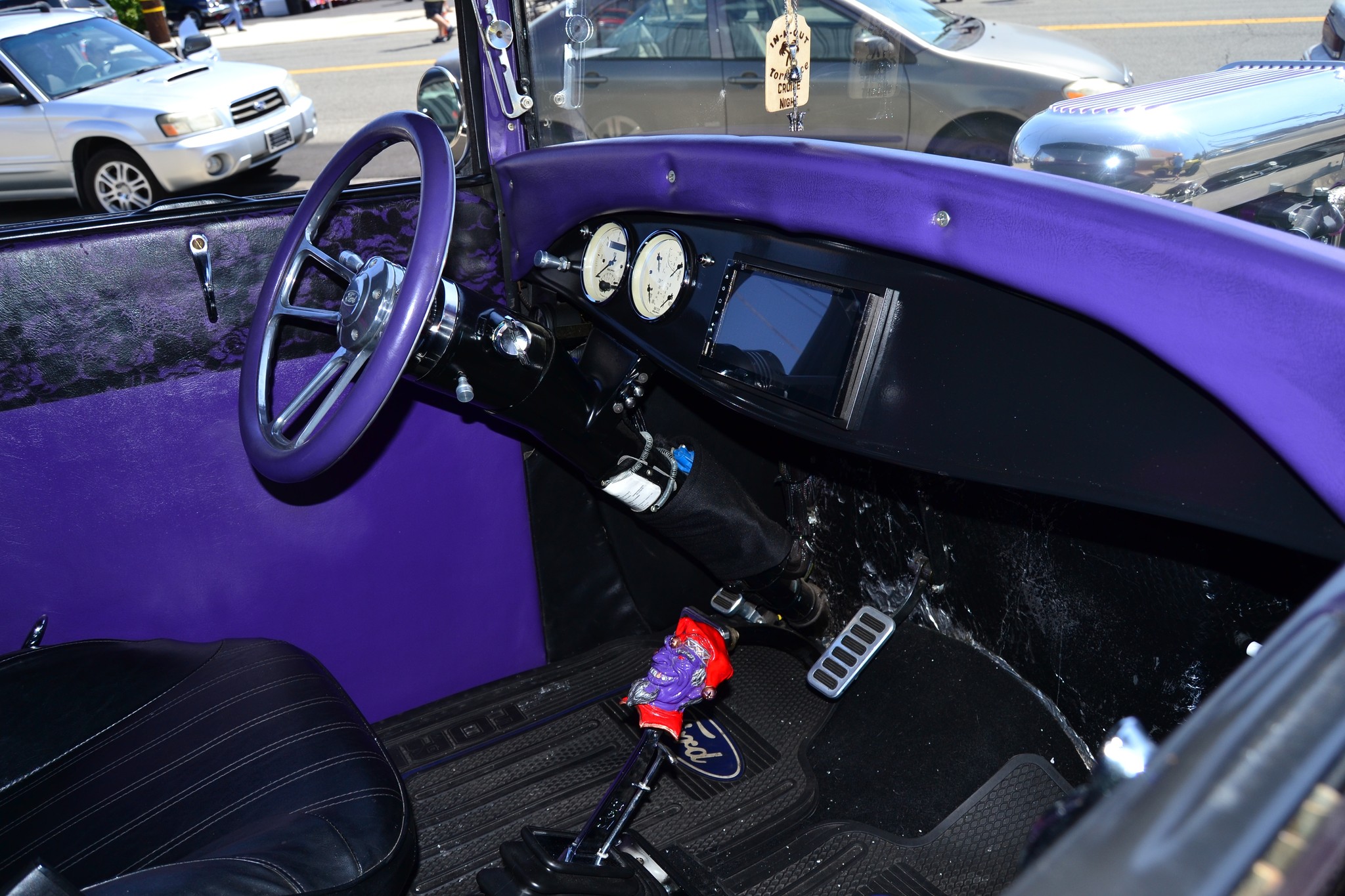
[0,67,1345,896]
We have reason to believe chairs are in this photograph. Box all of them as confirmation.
[19,48,75,90]
[0,631,423,896]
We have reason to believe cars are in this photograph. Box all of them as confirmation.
[433,0,1135,167]
[0,0,318,214]
[1009,1,1345,250]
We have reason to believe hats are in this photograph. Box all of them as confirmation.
[1326,0,1345,41]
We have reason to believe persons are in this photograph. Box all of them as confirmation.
[424,0,456,44]
[220,0,247,33]
[85,39,116,78]
[619,617,733,742]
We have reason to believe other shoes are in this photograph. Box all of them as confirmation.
[432,36,448,43]
[219,22,226,32]
[239,28,247,31]
[446,25,456,41]
